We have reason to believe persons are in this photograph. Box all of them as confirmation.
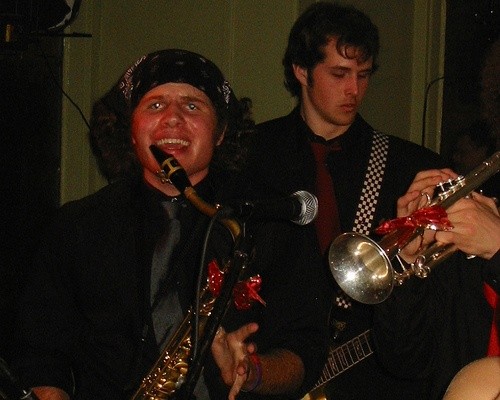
[239,1,450,400]
[396,163,498,400]
[0,47,327,400]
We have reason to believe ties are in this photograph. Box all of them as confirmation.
[150,200,212,400]
[309,142,342,256]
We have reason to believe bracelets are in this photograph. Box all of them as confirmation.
[241,352,261,394]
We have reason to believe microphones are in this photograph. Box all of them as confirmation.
[215,190,319,226]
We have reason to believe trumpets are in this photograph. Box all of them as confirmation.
[328,150,500,304]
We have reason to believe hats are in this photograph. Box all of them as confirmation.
[110,48,237,128]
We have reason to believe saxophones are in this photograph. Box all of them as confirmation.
[130,143,255,400]
